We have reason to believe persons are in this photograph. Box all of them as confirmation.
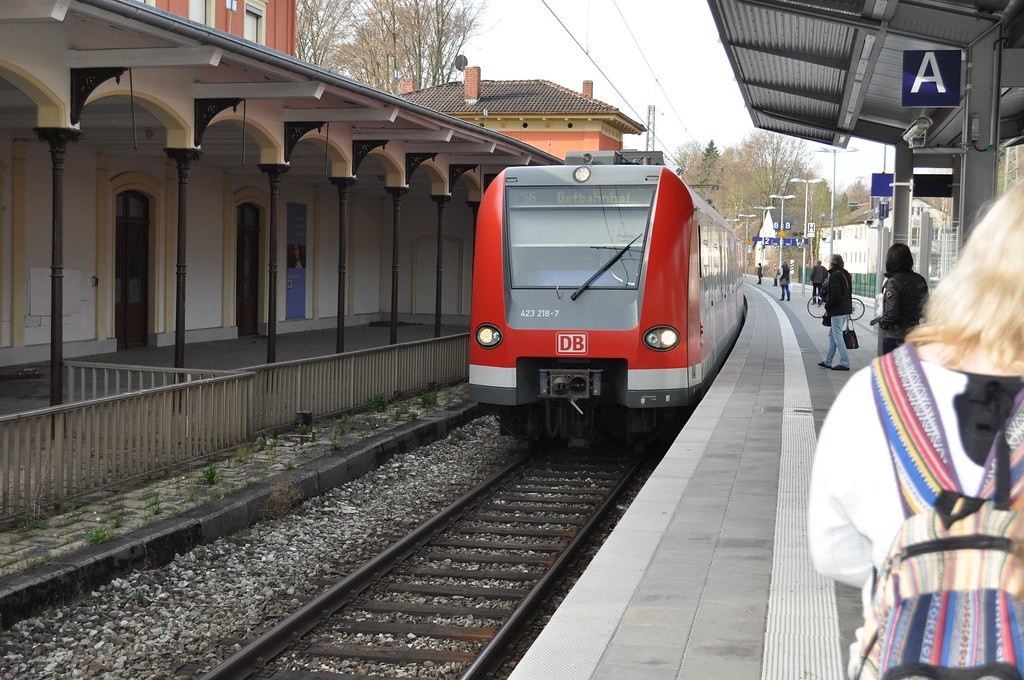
[772,264,778,286]
[807,182,1023,680]
[810,261,828,305]
[870,243,929,355]
[779,261,791,301]
[818,255,853,370]
[757,263,762,284]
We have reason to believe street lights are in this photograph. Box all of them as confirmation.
[769,193,797,287]
[752,205,777,274]
[738,213,758,278]
[813,146,859,302]
[789,178,825,297]
[725,218,741,227]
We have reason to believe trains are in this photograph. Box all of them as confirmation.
[465,147,745,445]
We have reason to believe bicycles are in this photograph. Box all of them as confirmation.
[807,294,865,321]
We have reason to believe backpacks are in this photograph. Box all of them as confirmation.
[854,343,1024,680]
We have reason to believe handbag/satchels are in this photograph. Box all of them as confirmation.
[780,279,786,286]
[759,273,763,277]
[822,314,831,326]
[842,315,859,349]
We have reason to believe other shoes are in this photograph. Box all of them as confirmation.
[831,365,850,370]
[787,299,790,301]
[818,362,831,368]
[779,298,784,300]
